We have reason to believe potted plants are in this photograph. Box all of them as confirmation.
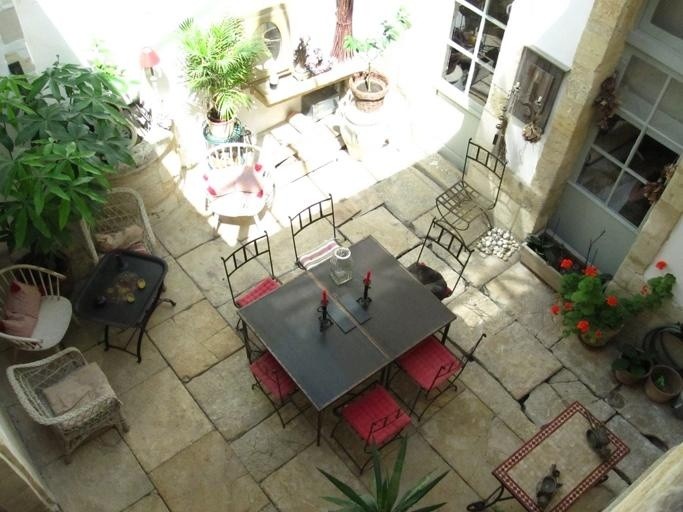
[612,342,658,385]
[343,3,412,112]
[0,53,140,300]
[519,217,612,296]
[176,12,274,139]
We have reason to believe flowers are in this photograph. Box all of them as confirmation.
[550,258,675,344]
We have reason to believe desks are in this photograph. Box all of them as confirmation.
[72,248,175,364]
[465,400,630,512]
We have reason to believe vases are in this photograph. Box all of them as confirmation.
[579,323,625,347]
[643,364,682,403]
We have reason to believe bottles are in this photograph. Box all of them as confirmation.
[328,247,354,286]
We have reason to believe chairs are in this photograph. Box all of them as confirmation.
[200,142,275,238]
[435,137,507,252]
